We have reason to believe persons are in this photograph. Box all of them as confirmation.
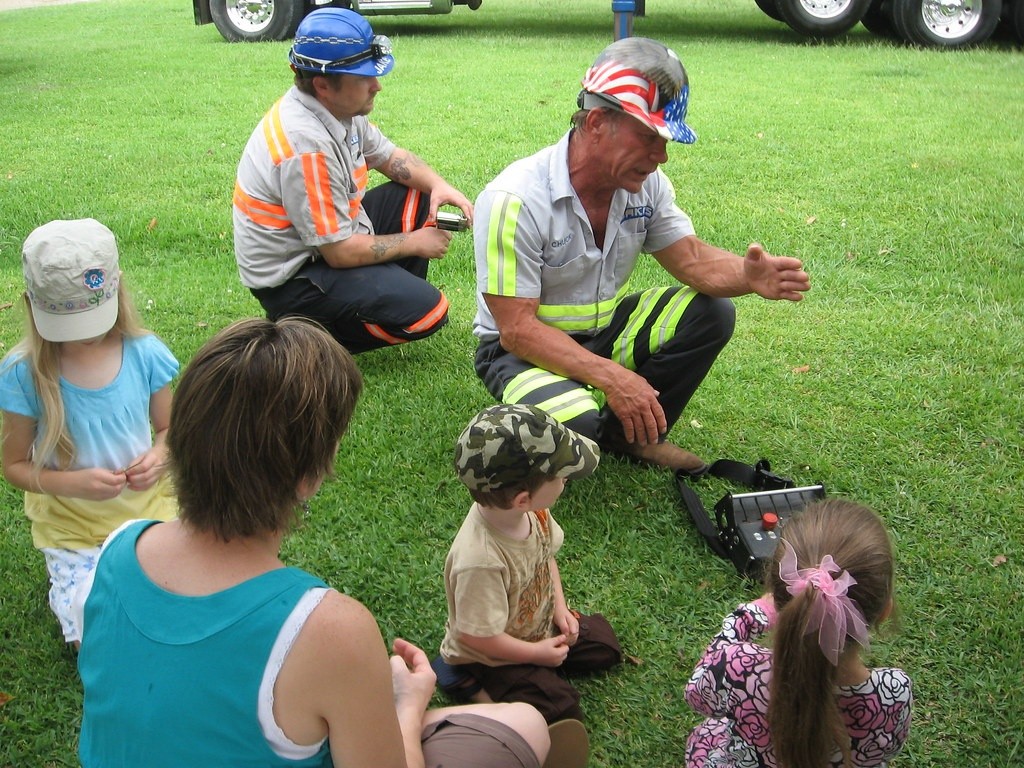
[233,8,472,355]
[75,316,550,768]
[684,498,914,768]
[473,37,810,479]
[429,404,622,727]
[0,218,179,656]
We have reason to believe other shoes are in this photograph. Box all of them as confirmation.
[599,431,708,477]
[540,718,589,768]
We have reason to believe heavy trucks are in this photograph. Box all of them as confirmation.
[755,0,1024,48]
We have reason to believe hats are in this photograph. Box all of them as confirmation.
[22,218,119,342]
[453,404,600,504]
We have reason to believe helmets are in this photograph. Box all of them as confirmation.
[577,36,697,144]
[289,7,394,77]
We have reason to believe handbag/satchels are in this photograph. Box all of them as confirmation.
[673,458,826,584]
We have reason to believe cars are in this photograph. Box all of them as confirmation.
[193,0,483,44]
